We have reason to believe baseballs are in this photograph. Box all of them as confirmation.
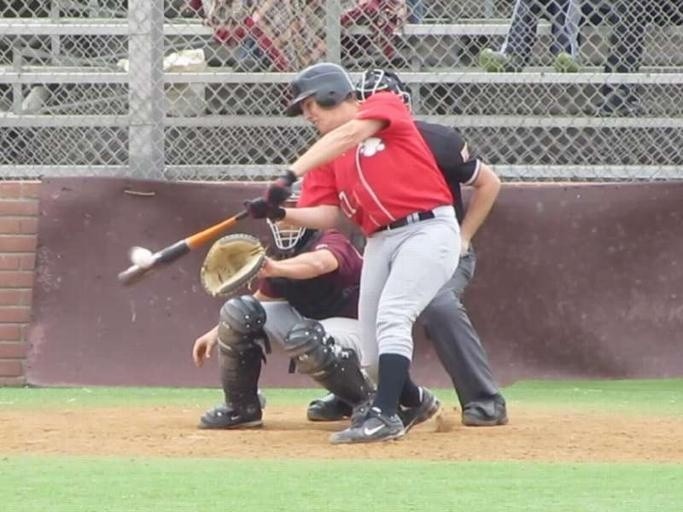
[130,247,151,264]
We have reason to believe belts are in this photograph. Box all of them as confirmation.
[371,203,457,233]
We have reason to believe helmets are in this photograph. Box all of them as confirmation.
[353,67,415,114]
[281,61,354,117]
[264,175,311,251]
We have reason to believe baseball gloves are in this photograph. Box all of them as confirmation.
[199,233,266,298]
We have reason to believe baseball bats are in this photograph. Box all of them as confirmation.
[118,209,249,286]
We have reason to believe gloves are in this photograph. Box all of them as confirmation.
[243,168,298,223]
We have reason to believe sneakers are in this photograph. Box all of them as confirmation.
[306,385,442,444]
[196,402,266,430]
[459,393,510,428]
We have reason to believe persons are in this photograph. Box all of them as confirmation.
[243,60,462,446]
[191,179,377,432]
[306,68,508,439]
[477,0,583,74]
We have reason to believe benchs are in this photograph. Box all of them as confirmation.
[0,19,682,182]
